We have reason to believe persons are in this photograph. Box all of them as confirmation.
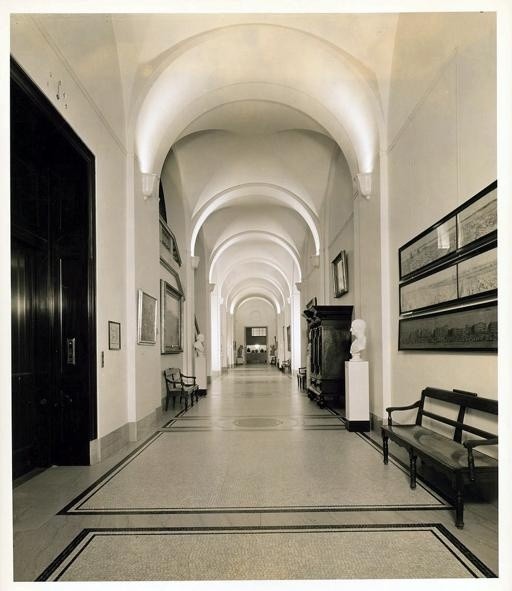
[194,334,205,353]
[349,319,366,354]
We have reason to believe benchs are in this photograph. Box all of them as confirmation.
[380,386,498,529]
[281,359,291,372]
[297,366,307,388]
[270,356,276,365]
[164,367,199,411]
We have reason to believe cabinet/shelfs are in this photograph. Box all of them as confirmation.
[301,305,354,409]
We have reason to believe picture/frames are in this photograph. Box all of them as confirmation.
[398,179,498,281]
[160,279,184,354]
[331,249,348,299]
[287,325,291,351]
[137,288,158,346]
[399,240,498,317]
[398,300,498,351]
[108,321,121,350]
